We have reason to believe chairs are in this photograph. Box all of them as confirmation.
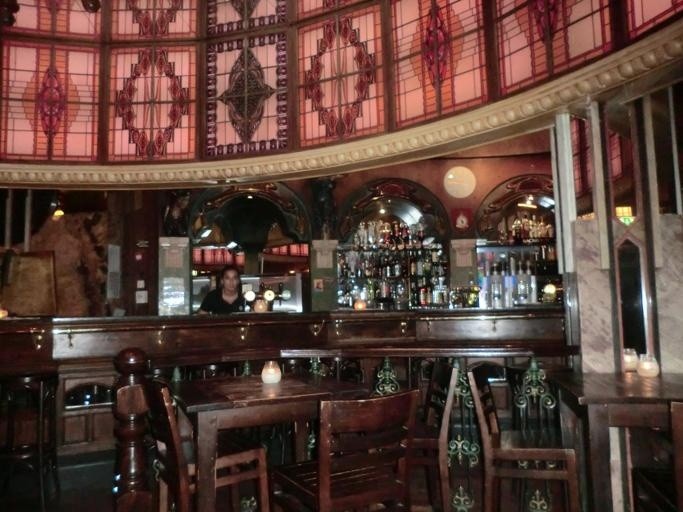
[631,400,683,512]
[367,362,458,511]
[269,387,421,511]
[467,362,581,511]
[144,379,270,512]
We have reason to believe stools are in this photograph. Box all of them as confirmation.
[2,365,62,511]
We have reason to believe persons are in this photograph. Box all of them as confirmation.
[198,266,253,314]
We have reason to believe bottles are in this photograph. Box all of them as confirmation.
[497,215,553,242]
[336,219,448,309]
[474,246,564,307]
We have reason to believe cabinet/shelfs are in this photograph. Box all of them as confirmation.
[476,243,562,282]
[339,247,449,302]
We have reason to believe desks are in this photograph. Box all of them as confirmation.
[167,376,371,512]
[544,371,683,512]
